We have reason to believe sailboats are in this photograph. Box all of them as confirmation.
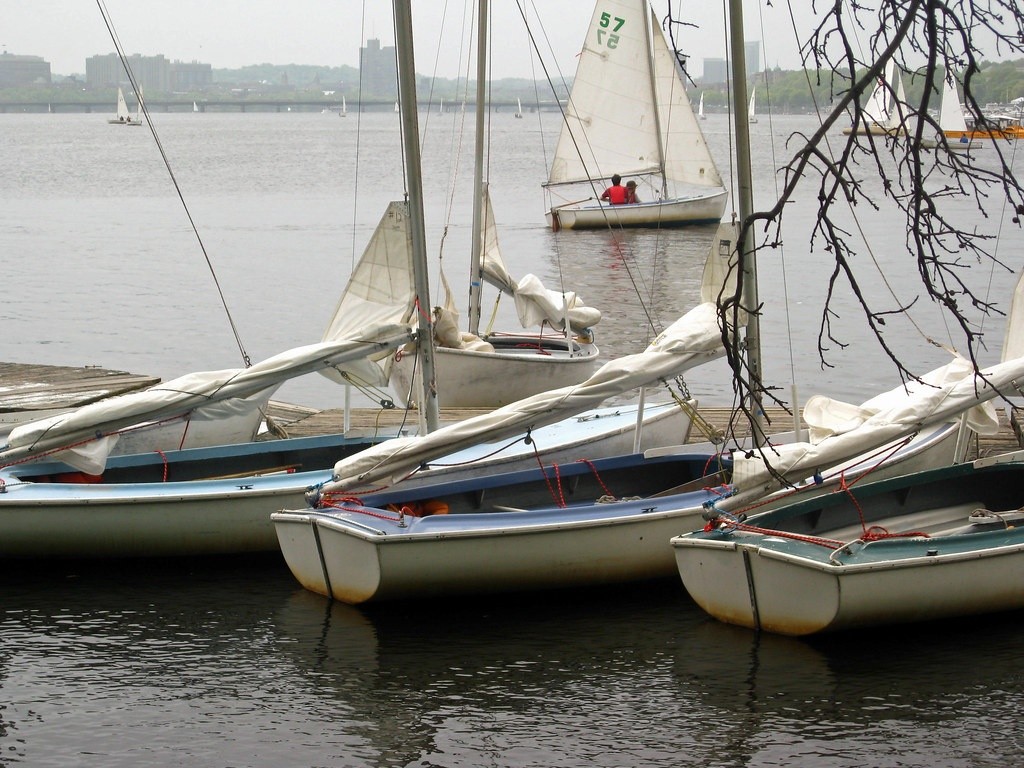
[541,0,729,230]
[438,98,443,117]
[919,43,983,149]
[338,95,346,118]
[0,0,746,567]
[699,92,707,120]
[842,52,913,136]
[107,86,129,124]
[268,0,1002,604]
[49,103,51,112]
[193,101,198,112]
[670,271,1024,637]
[376,0,601,409]
[126,82,144,126]
[394,102,399,114]
[514,98,523,118]
[747,85,758,123]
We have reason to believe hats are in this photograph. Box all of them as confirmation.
[627,181,638,189]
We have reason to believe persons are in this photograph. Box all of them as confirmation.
[600,174,628,205]
[624,180,642,204]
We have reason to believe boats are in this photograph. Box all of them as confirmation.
[942,114,1024,138]
